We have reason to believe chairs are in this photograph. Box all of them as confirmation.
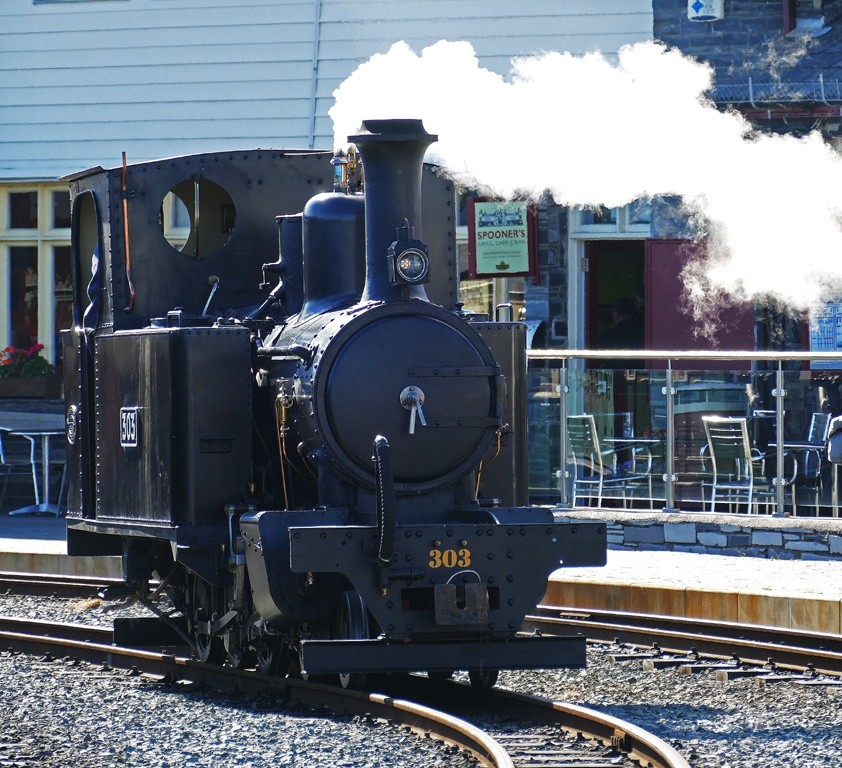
[562,414,654,511]
[699,412,834,518]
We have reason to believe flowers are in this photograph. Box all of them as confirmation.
[0,344,53,380]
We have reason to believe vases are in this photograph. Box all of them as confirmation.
[0,376,50,397]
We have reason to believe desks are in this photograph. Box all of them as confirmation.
[602,437,660,511]
[766,441,826,516]
[0,430,67,516]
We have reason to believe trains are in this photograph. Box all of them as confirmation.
[56,118,609,690]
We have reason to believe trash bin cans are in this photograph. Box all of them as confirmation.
[674,382,749,511]
